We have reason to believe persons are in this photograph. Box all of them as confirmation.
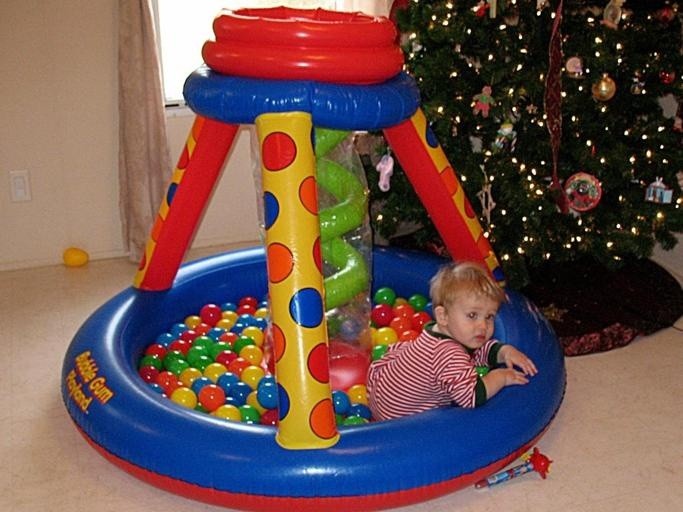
[369,263,540,423]
